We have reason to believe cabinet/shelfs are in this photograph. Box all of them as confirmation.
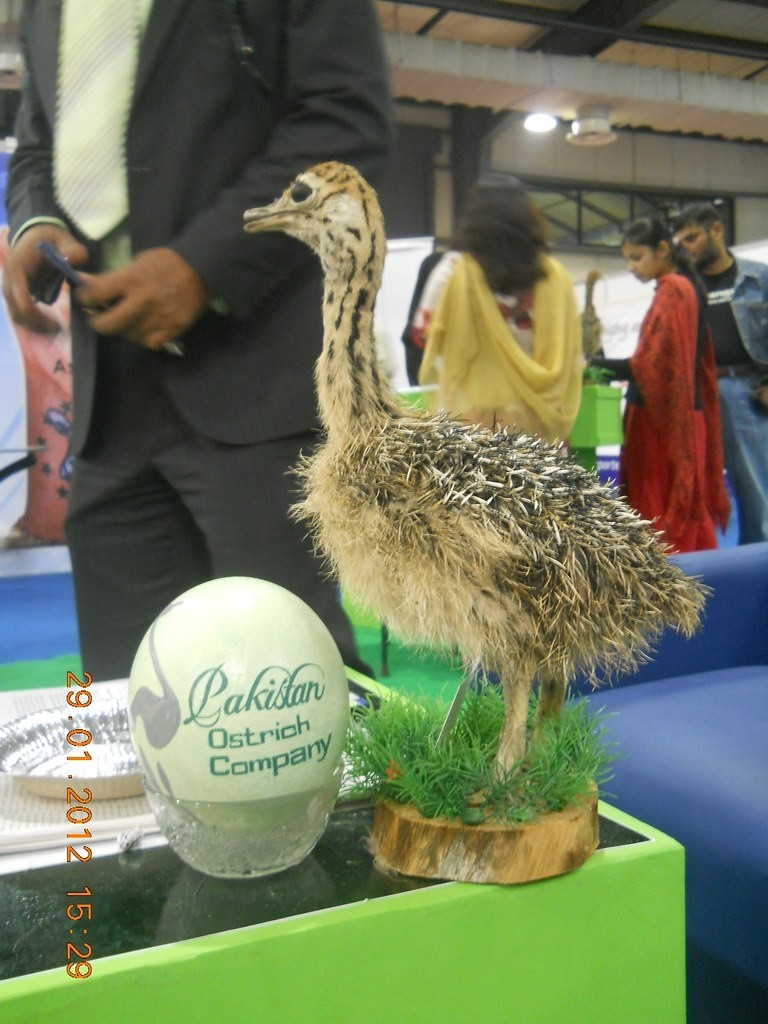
[0,658,688,1024]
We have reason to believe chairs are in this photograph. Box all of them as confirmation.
[472,540,768,1024]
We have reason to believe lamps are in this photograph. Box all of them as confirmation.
[565,98,618,146]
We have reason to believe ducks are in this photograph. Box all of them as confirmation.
[242,160,717,809]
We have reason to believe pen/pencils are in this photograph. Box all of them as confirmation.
[38,243,183,358]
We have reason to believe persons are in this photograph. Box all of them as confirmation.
[402,233,453,387]
[1,1,397,704]
[589,217,732,555]
[416,175,584,458]
[671,206,768,545]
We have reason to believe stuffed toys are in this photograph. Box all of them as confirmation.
[241,161,715,781]
